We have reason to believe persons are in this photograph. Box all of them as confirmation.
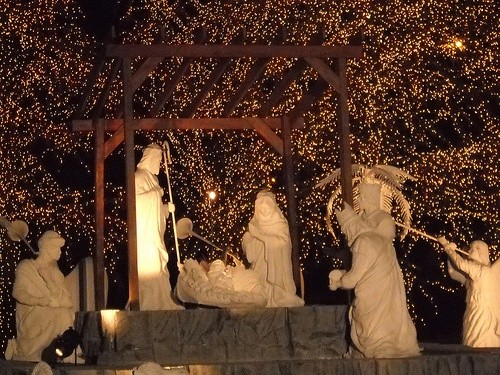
[4,230,85,363]
[30,361,53,375]
[125,146,187,311]
[330,203,422,358]
[207,260,234,290]
[358,183,396,242]
[133,362,168,375]
[438,237,500,347]
[242,190,305,308]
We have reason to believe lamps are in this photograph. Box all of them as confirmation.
[54,329,81,360]
